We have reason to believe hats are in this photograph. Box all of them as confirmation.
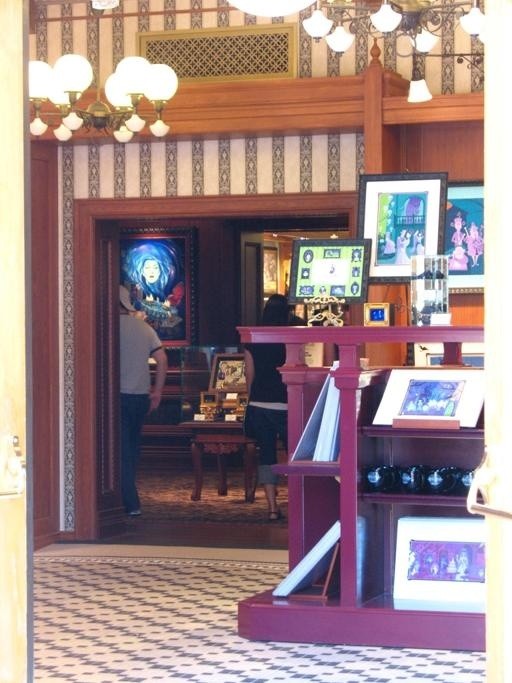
[118,286,136,312]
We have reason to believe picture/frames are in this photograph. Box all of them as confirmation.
[119,225,198,350]
[287,172,485,305]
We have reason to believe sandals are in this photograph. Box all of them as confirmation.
[269,508,281,521]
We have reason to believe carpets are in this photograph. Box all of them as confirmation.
[35,554,484,681]
[121,464,292,529]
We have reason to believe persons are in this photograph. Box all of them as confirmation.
[118,284,170,515]
[243,293,294,521]
[140,257,168,302]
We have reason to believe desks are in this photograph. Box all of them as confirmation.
[177,419,264,504]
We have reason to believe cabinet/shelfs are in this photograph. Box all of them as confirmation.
[225,327,484,655]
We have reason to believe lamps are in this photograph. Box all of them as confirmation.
[25,52,180,145]
[297,0,485,106]
